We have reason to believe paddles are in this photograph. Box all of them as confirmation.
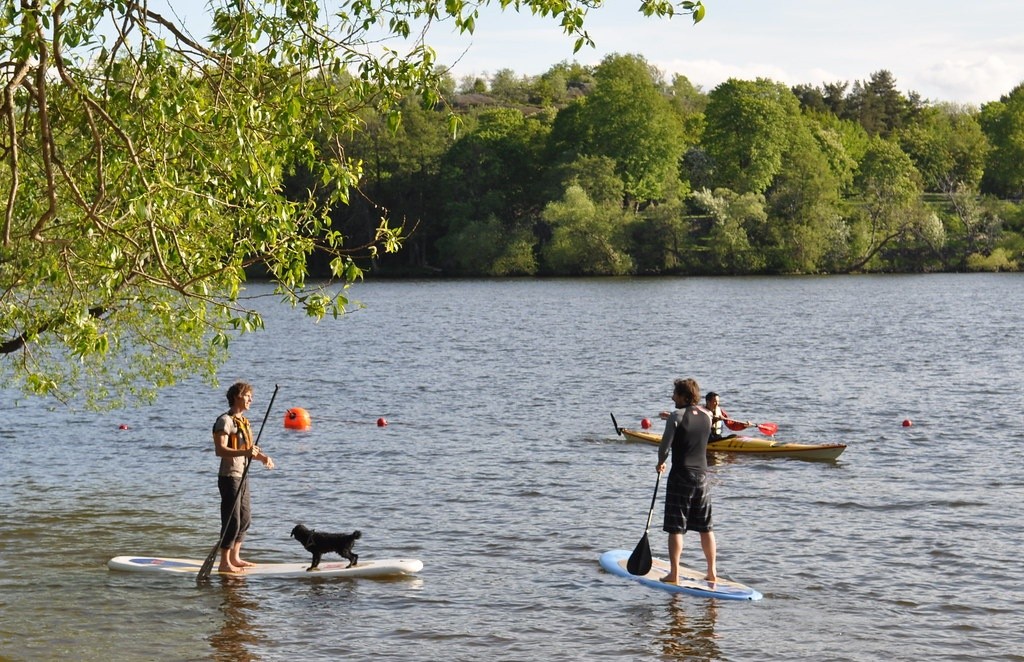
[195,384,281,585]
[712,415,777,436]
[626,472,662,576]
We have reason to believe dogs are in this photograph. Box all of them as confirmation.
[290,524,362,572]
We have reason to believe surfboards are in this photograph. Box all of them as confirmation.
[105,556,426,581]
[598,548,765,602]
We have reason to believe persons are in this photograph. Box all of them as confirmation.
[212,381,274,575]
[702,391,752,441]
[654,379,718,584]
